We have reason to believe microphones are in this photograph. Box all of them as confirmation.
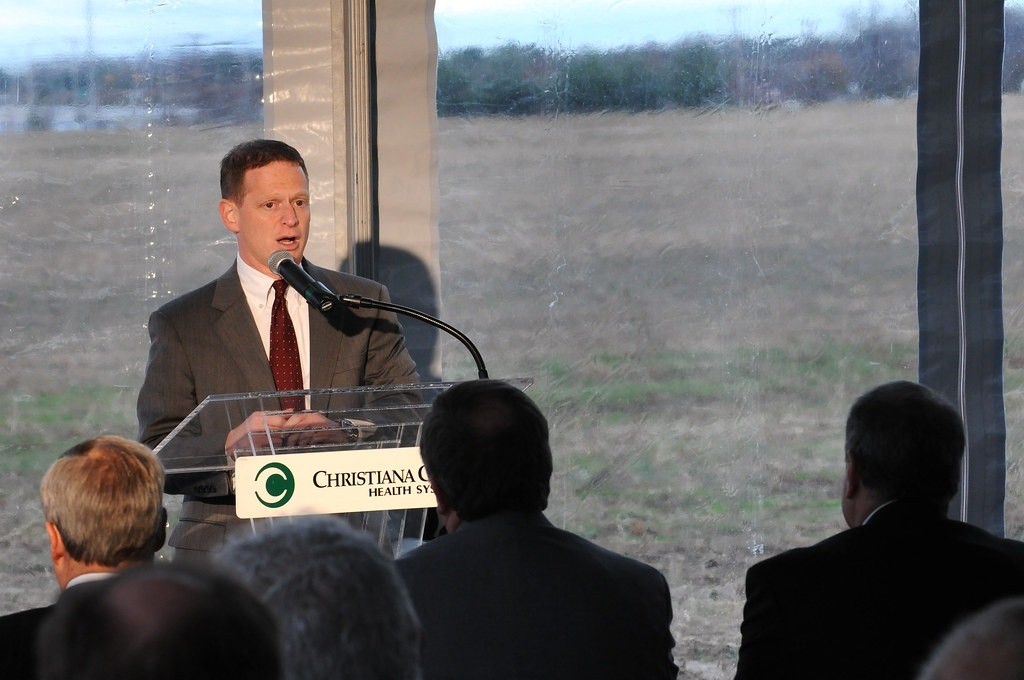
[268,250,332,312]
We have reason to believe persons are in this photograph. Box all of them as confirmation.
[915,598,1024,680]
[734,381,1024,680]
[31,561,286,680]
[0,435,170,680]
[386,379,682,680]
[134,140,427,564]
[209,512,422,680]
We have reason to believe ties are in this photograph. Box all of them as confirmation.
[269,278,307,419]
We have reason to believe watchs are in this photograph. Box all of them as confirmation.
[336,418,357,443]
